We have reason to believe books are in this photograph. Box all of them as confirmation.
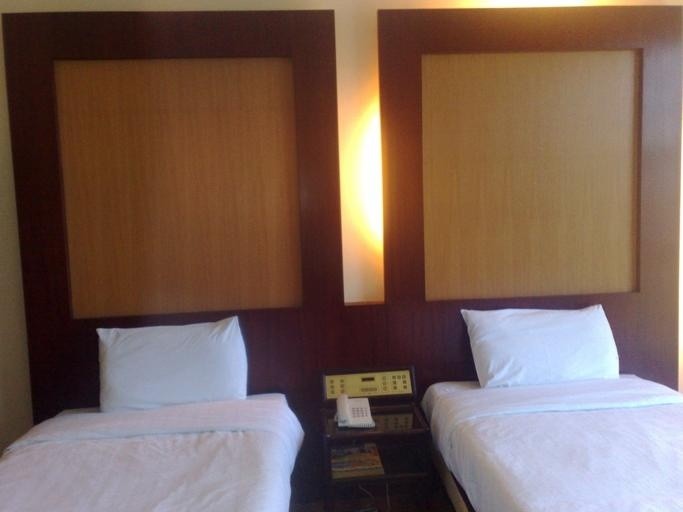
[330,440,385,480]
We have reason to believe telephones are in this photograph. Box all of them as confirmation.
[336,394,373,428]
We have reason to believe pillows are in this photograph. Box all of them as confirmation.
[96,316,247,413]
[461,304,619,388]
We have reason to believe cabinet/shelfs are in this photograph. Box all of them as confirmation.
[307,364,431,512]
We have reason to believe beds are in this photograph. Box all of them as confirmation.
[420,372,674,511]
[2,391,304,512]
